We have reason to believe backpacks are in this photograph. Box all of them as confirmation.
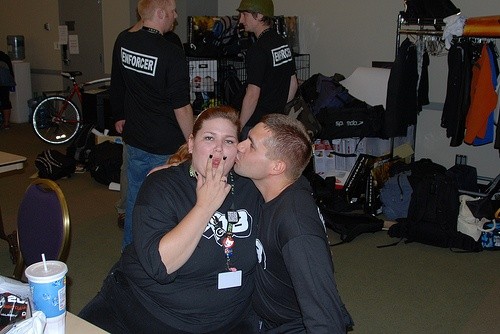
[88,140,124,186]
[375,168,413,222]
[387,157,461,248]
[299,72,367,117]
[34,148,74,180]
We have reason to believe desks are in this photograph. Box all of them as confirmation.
[0,275,110,334]
[0,151,27,240]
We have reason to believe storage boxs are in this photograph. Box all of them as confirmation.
[314,126,414,173]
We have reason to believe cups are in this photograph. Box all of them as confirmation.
[24,260,69,334]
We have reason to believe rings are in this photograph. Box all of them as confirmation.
[221,180,227,184]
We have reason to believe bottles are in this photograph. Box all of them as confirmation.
[29,92,53,129]
[7,35,25,60]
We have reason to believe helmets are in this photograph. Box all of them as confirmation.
[235,0,274,19]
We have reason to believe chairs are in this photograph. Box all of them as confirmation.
[9,178,70,279]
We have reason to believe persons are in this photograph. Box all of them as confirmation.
[76,106,264,334]
[237,0,298,141]
[109,9,184,231]
[122,0,196,257]
[147,114,354,334]
[0,50,16,130]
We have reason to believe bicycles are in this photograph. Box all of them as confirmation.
[31,70,111,146]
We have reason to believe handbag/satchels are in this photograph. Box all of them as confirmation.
[314,105,387,139]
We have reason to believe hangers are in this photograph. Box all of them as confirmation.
[406,29,500,58]
